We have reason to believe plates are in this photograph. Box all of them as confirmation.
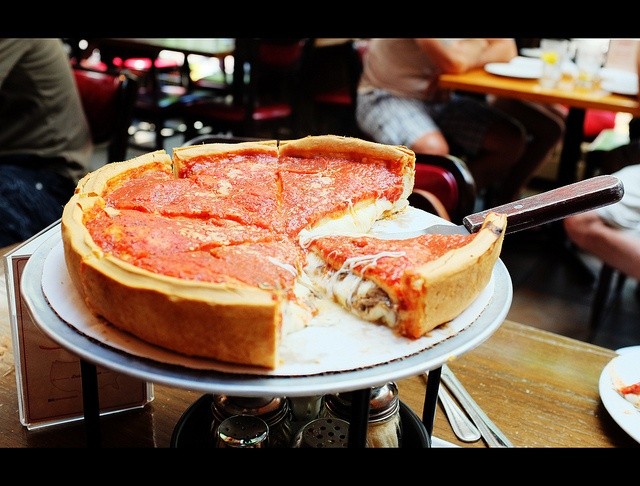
[483,62,542,78]
[599,347,640,444]
[17,203,513,398]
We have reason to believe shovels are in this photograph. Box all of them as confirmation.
[294,176,624,249]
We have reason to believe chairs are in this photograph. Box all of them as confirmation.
[289,40,476,224]
[180,135,477,225]
[69,65,141,165]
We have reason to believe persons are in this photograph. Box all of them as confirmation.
[353,36,518,158]
[562,163,640,284]
[0,37,91,252]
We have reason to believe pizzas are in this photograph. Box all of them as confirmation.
[63,135,415,369]
[304,212,507,340]
